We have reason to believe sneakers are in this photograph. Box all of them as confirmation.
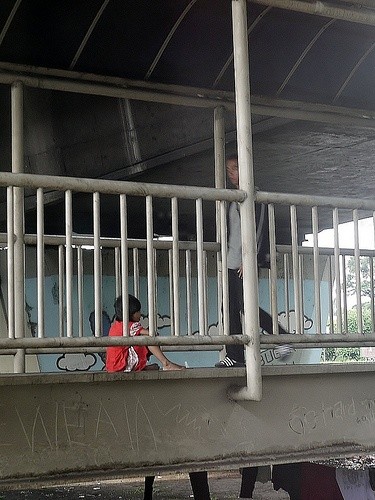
[215,356,245,367]
[279,344,296,362]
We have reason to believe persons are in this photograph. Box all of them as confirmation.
[104,293,186,373]
[212,154,296,367]
